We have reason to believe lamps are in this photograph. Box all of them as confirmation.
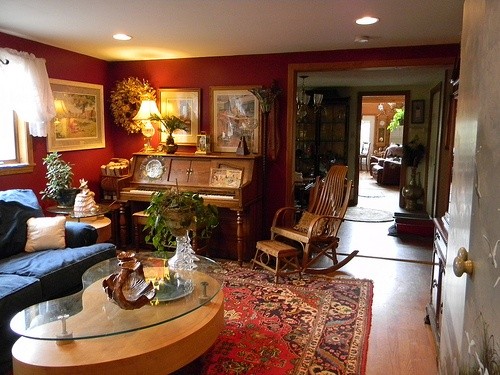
[247,87,280,265]
[295,75,323,123]
[132,99,162,153]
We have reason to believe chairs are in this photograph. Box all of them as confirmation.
[360,143,370,172]
[271,164,360,284]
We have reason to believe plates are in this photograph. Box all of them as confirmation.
[146,159,163,178]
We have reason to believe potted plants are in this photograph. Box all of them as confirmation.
[401,134,426,210]
[38,151,78,208]
[143,178,219,270]
[147,113,189,154]
[386,108,404,131]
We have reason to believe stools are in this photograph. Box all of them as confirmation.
[97,203,129,243]
[251,241,303,283]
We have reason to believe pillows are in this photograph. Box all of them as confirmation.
[24,216,67,252]
[295,210,328,236]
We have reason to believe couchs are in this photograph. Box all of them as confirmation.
[0,188,117,364]
[371,145,402,185]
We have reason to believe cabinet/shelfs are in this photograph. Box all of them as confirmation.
[423,77,462,356]
[295,96,352,204]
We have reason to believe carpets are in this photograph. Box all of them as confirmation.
[200,258,373,375]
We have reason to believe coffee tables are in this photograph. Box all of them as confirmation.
[10,252,224,375]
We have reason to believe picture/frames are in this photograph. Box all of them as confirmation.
[211,85,263,153]
[411,99,425,124]
[158,87,201,146]
[46,77,105,151]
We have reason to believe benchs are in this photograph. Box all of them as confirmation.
[132,211,211,257]
